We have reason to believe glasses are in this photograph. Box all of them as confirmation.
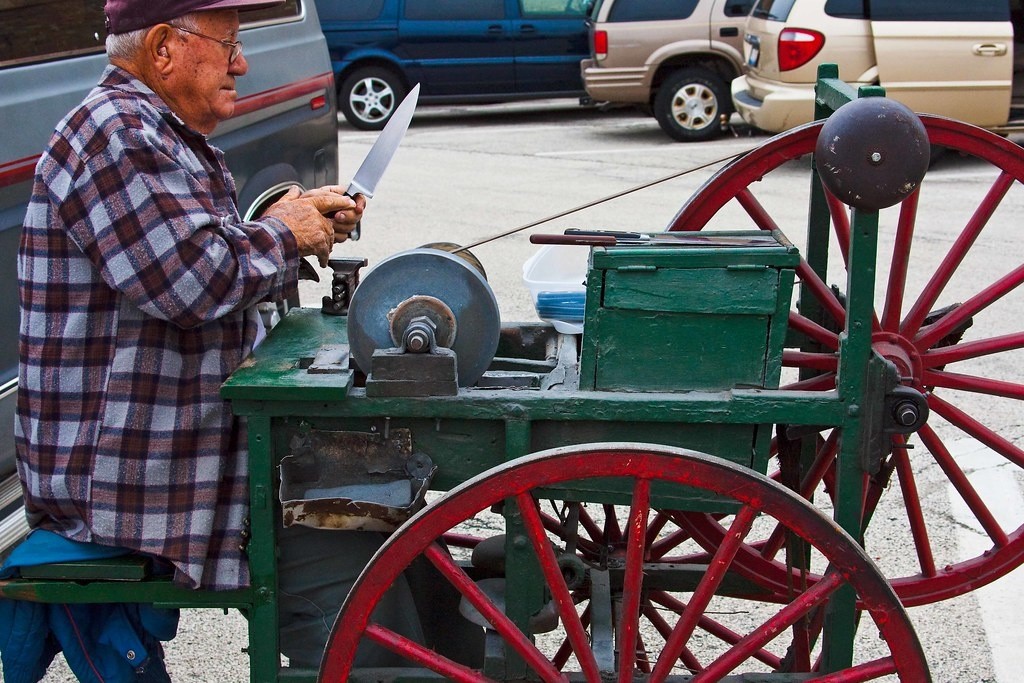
[169,20,243,62]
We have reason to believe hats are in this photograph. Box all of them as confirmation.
[104,0,285,33]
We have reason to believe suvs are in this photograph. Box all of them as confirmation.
[580,0,757,142]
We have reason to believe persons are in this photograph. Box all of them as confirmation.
[13,0,486,669]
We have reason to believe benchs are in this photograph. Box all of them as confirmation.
[2,526,170,581]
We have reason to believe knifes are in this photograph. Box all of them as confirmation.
[564,227,777,244]
[530,234,782,247]
[331,81,421,219]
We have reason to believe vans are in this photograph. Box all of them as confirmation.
[730,0,1024,135]
[0,0,339,555]
[314,0,595,131]
